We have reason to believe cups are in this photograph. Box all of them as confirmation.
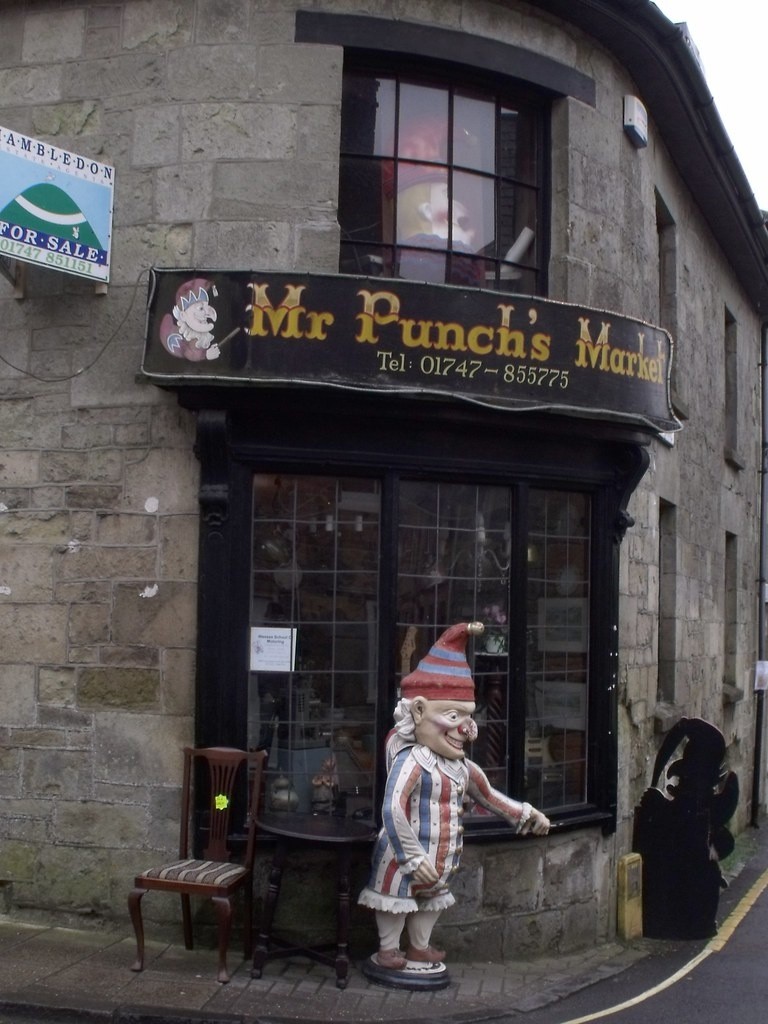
[485,636,505,654]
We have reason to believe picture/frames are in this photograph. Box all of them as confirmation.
[538,596,589,652]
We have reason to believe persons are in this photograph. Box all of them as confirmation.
[375,113,496,289]
[360,623,550,963]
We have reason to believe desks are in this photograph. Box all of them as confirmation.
[245,813,377,989]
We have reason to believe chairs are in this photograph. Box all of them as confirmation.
[128,746,267,983]
